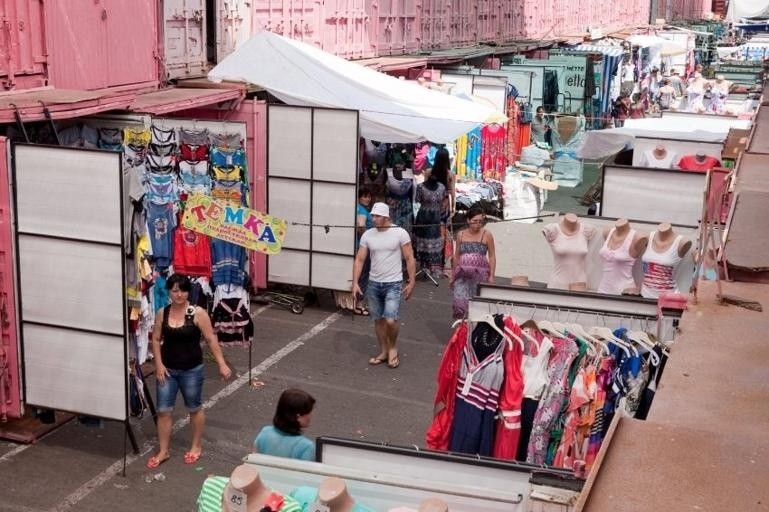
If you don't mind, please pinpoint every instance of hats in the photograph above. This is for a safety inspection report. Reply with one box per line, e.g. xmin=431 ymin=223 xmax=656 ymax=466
xmin=370 ymin=202 xmax=389 ymax=218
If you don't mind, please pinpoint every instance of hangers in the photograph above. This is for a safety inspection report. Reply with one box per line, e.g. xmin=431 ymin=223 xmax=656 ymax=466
xmin=148 ymin=118 xmax=245 ymax=211
xmin=448 ymin=299 xmax=673 ymax=366
xmin=70 ymin=121 xmax=147 ymax=152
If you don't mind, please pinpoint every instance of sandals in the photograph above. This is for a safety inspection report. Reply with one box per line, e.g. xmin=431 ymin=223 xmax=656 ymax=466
xmin=354 ymin=307 xmax=369 ymax=315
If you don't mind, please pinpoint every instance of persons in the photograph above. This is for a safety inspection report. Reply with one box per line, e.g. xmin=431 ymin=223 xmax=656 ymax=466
xmin=352 ymin=137 xmax=495 ymax=368
xmin=147 ymin=273 xmax=231 ymax=468
xmin=637 ymin=144 xmax=680 ymax=169
xmin=511 ymin=212 xmax=693 ymax=298
xmin=197 ymin=466 xmax=448 ymax=512
xmin=677 ymin=152 xmax=722 ymax=171
xmin=686 ymin=73 xmax=711 ymax=113
xmin=530 ymin=106 xmax=549 ymax=144
xmin=613 ymin=50 xmax=703 ymax=128
xmin=707 ymin=74 xmax=734 ymax=115
xmin=253 ymin=389 xmax=317 ymax=460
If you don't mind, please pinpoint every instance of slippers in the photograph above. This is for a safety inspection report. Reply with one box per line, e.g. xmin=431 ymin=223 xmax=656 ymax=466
xmin=147 ymin=457 xmax=160 ymax=470
xmin=183 ymin=452 xmax=201 ymax=464
xmin=368 ymin=352 xmax=400 ymax=367
